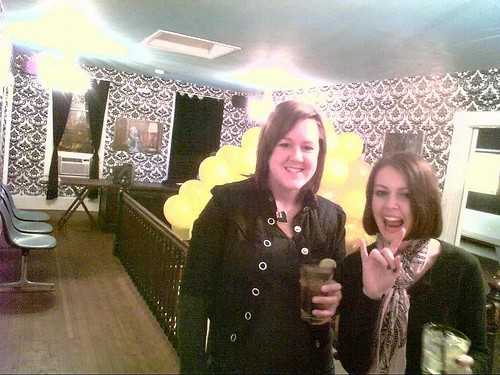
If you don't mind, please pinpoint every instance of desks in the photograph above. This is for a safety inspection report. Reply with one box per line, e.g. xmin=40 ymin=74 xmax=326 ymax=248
xmin=59 ymin=177 xmax=113 ymax=231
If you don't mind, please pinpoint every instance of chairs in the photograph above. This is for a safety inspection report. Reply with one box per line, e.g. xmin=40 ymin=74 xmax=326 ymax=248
xmin=0 ymin=183 xmax=57 ymax=290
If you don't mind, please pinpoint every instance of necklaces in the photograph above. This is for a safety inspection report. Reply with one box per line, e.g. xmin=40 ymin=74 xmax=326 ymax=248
xmin=275 ymin=203 xmax=298 ymax=225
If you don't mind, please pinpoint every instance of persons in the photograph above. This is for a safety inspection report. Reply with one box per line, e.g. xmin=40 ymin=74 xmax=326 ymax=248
xmin=176 ymin=100 xmax=347 ymax=375
xmin=337 ymin=152 xmax=488 ymax=375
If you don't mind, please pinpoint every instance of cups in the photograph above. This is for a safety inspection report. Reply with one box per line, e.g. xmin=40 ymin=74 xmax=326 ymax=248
xmin=420 ymin=324 xmax=471 ymax=375
xmin=299 ymin=264 xmax=334 ymax=323
